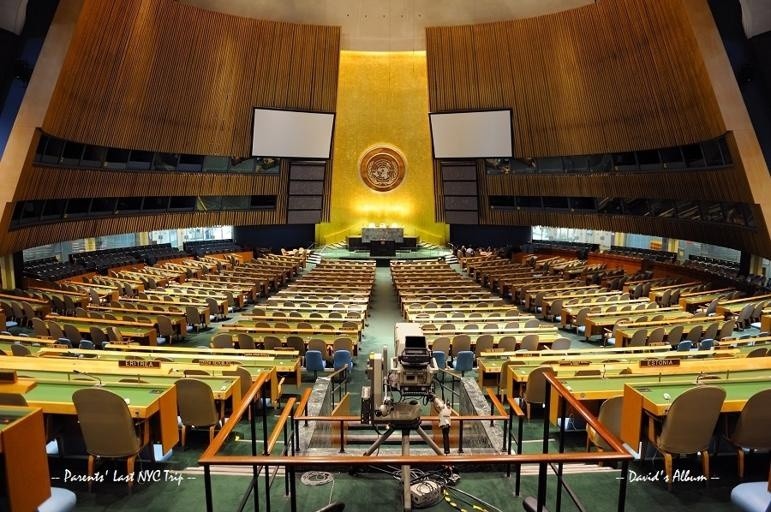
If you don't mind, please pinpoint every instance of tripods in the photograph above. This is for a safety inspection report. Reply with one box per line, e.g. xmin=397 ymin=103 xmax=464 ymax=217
xmin=348 ymin=427 xmax=447 ymax=512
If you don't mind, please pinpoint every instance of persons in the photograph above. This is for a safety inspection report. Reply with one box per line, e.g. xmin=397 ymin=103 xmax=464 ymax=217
xmin=460 ymin=244 xmax=499 ymax=258
